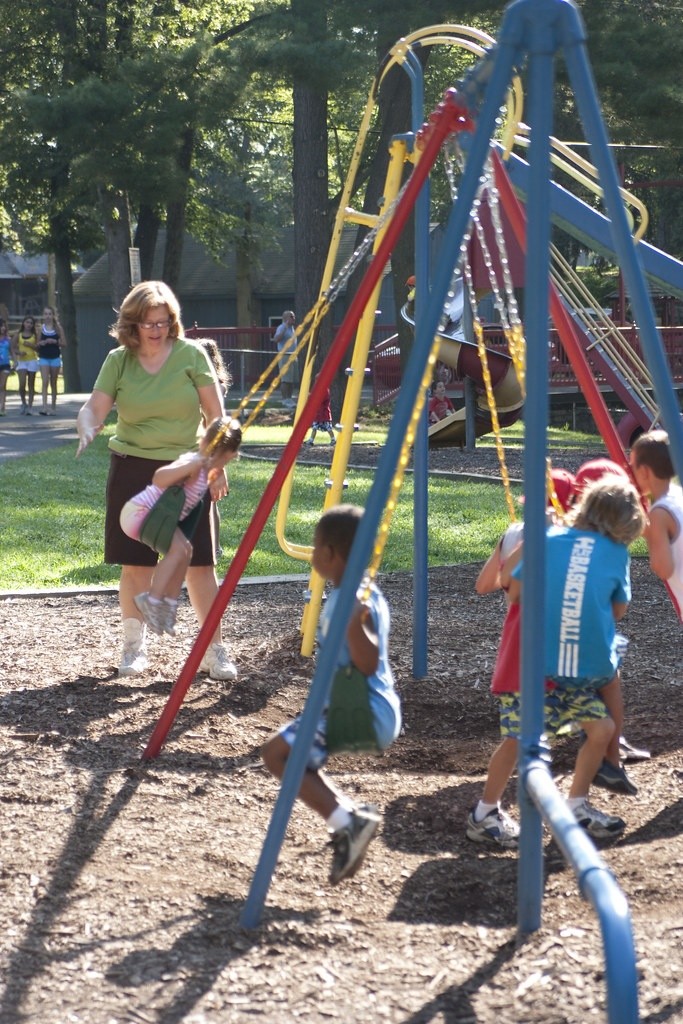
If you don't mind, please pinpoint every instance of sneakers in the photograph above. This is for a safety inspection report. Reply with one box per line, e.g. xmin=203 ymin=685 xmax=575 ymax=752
xmin=118 ymin=634 xmax=147 ymax=675
xmin=594 ymin=758 xmax=638 ymax=796
xmin=465 ymin=806 xmax=520 ymax=850
xmin=165 ymin=601 xmax=179 ymax=638
xmin=572 ymin=795 xmax=626 ymax=838
xmin=326 ymin=804 xmax=381 ymax=888
xmin=201 ymin=643 xmax=237 ymax=680
xmin=133 ymin=593 xmax=168 ymax=637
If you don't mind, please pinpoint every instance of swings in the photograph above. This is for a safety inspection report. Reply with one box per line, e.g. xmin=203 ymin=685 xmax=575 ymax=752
xmin=318 ymin=90 xmax=569 ymax=759
xmin=137 ymin=161 xmax=418 ymax=556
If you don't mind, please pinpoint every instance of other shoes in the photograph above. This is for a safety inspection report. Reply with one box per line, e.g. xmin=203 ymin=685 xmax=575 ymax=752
xmin=26 ymin=408 xmax=33 ymax=415
xmin=617 ymin=736 xmax=651 ymax=762
xmin=0 ymin=410 xmax=6 ymax=416
xmin=304 ymin=440 xmax=314 ymax=445
xmin=20 ymin=405 xmax=27 ymax=414
xmin=330 ymin=439 xmax=337 ymax=445
xmin=282 ymin=399 xmax=296 ymax=408
xmin=39 ymin=408 xmax=56 ymax=415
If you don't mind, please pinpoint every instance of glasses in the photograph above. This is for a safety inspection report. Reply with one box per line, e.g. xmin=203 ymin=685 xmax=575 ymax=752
xmin=287 ymin=316 xmax=296 ymax=319
xmin=137 ymin=319 xmax=172 ymax=328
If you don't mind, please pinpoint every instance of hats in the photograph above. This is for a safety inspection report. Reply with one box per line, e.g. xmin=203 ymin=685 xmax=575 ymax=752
xmin=405 ymin=275 xmax=415 ymax=285
xmin=575 ymin=458 xmax=630 ymax=502
xmin=517 ymin=468 xmax=579 ymax=512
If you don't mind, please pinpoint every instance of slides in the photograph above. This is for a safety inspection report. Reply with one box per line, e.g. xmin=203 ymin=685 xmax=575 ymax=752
xmin=399 ymin=275 xmax=528 ymax=448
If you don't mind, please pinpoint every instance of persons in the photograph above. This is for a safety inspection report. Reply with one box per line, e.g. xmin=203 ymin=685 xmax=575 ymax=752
xmin=0 ymin=318 xmax=18 ymax=416
xmin=36 ymin=306 xmax=67 ymax=416
xmin=428 ymin=382 xmax=456 ymax=425
xmin=405 ymin=276 xmax=416 ymax=301
xmin=76 ymin=281 xmax=239 ymax=680
xmin=273 ymin=310 xmax=300 ymax=408
xmin=12 ymin=316 xmax=39 ymax=415
xmin=303 ymin=374 xmax=335 ymax=446
xmin=195 ymin=339 xmax=231 ymax=434
xmin=467 ymin=430 xmax=683 ymax=847
xmin=263 ymin=507 xmax=403 ymax=887
xmin=120 ymin=416 xmax=242 ymax=635
